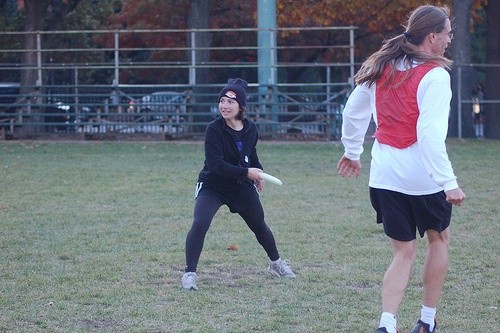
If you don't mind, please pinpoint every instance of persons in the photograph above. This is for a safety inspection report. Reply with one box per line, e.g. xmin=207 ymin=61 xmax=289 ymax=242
xmin=470 ymin=83 xmax=488 ymax=138
xmin=337 ymin=6 xmax=465 ymax=333
xmin=181 ymin=79 xmax=296 ymax=292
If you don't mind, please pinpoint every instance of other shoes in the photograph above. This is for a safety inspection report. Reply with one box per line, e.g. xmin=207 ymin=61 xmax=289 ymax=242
xmin=412 ymin=319 xmax=439 ymax=333
xmin=376 ymin=326 xmax=401 ymax=333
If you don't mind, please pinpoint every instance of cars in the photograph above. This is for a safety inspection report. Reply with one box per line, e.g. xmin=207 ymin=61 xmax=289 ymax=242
xmin=212 ymin=93 xmax=344 ymax=132
xmin=138 ymin=92 xmax=185 ymax=121
xmin=0 ymin=83 xmax=88 ymax=135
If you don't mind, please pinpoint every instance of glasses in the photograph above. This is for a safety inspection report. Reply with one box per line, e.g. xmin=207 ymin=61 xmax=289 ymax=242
xmin=435 ymin=33 xmax=454 ymax=39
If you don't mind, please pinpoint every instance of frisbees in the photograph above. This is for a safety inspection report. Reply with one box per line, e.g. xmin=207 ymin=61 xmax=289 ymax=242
xmin=260 ymin=172 xmax=283 ymax=186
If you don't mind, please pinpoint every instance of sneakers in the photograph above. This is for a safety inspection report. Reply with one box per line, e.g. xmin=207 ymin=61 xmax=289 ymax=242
xmin=181 ymin=272 xmax=199 ymax=291
xmin=267 ymin=258 xmax=297 ymax=281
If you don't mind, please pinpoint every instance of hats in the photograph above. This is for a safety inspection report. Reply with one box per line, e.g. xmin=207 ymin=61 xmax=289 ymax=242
xmin=218 ymin=78 xmax=249 ymax=109
